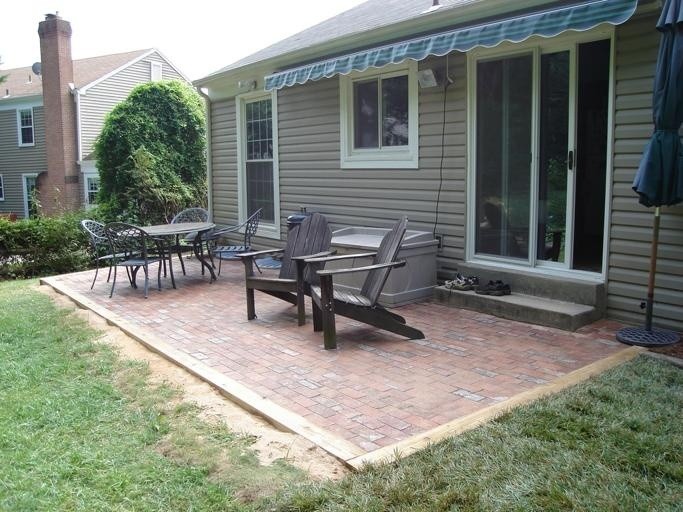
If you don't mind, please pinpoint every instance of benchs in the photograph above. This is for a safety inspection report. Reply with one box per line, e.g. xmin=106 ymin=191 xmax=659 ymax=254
xmin=324 ymin=226 xmax=439 ymax=309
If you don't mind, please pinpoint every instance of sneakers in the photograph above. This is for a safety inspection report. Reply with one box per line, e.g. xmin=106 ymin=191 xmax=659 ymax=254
xmin=476 ymin=280 xmax=511 ymax=296
xmin=444 ymin=274 xmax=480 ymax=291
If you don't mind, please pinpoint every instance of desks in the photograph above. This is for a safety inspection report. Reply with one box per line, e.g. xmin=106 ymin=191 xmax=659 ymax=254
xmin=117 ymin=222 xmax=216 ymax=292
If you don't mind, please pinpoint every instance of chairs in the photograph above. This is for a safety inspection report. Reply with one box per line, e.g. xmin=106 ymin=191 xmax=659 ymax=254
xmin=209 ymin=208 xmax=264 ymax=284
xmin=81 ymin=220 xmax=139 ymax=299
xmin=479 ymin=203 xmax=566 ymax=261
xmin=104 ymin=222 xmax=176 ymax=300
xmin=304 ymin=214 xmax=424 ymax=351
xmin=234 ymin=213 xmax=332 ymax=327
xmin=162 ymin=208 xmax=216 ymax=275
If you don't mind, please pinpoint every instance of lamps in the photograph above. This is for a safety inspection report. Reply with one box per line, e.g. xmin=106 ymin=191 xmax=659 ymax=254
xmin=416 ymin=68 xmax=438 ymax=89
xmin=237 ymin=79 xmax=257 ymax=90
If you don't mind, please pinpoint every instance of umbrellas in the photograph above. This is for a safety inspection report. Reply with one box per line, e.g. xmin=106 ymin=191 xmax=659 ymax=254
xmin=630 ymin=1 xmax=682 ymax=333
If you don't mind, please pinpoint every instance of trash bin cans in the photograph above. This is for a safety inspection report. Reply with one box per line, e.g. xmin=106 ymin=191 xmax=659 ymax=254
xmin=287 ymin=214 xmax=308 ymax=232
